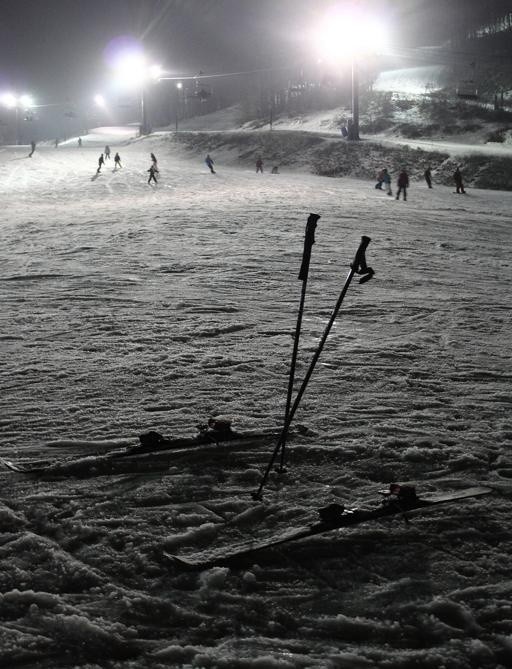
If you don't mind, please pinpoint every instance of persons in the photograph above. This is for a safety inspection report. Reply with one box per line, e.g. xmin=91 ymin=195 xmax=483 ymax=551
xmin=383 ymin=169 xmax=392 ymax=195
xmin=114 ymin=152 xmax=123 ymax=170
xmin=97 ymin=153 xmax=106 ymax=173
xmin=452 ymin=166 xmax=466 ymax=194
xmin=395 ymin=169 xmax=410 ymax=201
xmin=255 ymin=157 xmax=264 ymax=173
xmin=78 ymin=137 xmax=82 ymax=146
xmin=150 ymin=153 xmax=158 ymax=170
xmin=55 ymin=137 xmax=59 ymax=148
xmin=104 ymin=145 xmax=111 ymax=160
xmin=147 ymin=166 xmax=160 ymax=184
xmin=374 ymin=169 xmax=385 ymax=189
xmin=424 ymin=166 xmax=432 ymax=188
xmin=29 ymin=140 xmax=36 ymax=157
xmin=205 ymin=154 xmax=217 ymax=173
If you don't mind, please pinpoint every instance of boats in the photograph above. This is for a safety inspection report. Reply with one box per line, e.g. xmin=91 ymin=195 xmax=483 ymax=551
xmin=165 ymin=482 xmax=495 ymax=567
xmin=5 ymin=422 xmax=310 ymax=475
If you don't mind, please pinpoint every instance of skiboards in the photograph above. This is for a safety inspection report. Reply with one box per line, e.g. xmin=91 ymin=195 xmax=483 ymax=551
xmin=2 ymin=423 xmax=493 ymax=567
